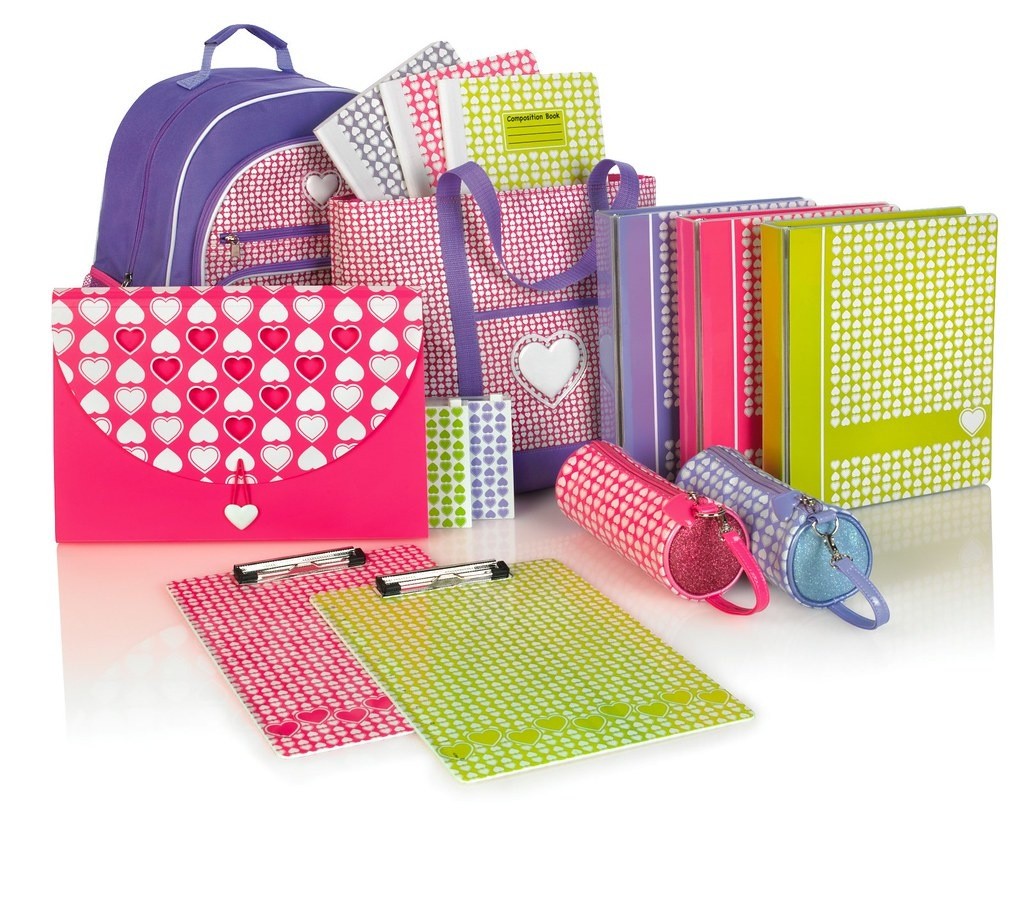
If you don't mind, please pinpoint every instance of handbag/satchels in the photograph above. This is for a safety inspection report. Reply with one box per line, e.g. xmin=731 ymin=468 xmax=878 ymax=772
xmin=328 ymin=159 xmax=656 ymax=494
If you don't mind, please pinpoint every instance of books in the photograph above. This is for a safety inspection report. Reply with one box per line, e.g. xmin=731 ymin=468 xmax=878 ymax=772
xmin=425 ymin=393 xmax=515 ymax=527
xmin=312 ymin=40 xmax=607 ymax=200
xmin=591 ymin=192 xmax=998 ymax=509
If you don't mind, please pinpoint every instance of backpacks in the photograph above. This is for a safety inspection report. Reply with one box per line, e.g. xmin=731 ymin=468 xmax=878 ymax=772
xmin=92 ymin=24 xmax=362 ymax=285
